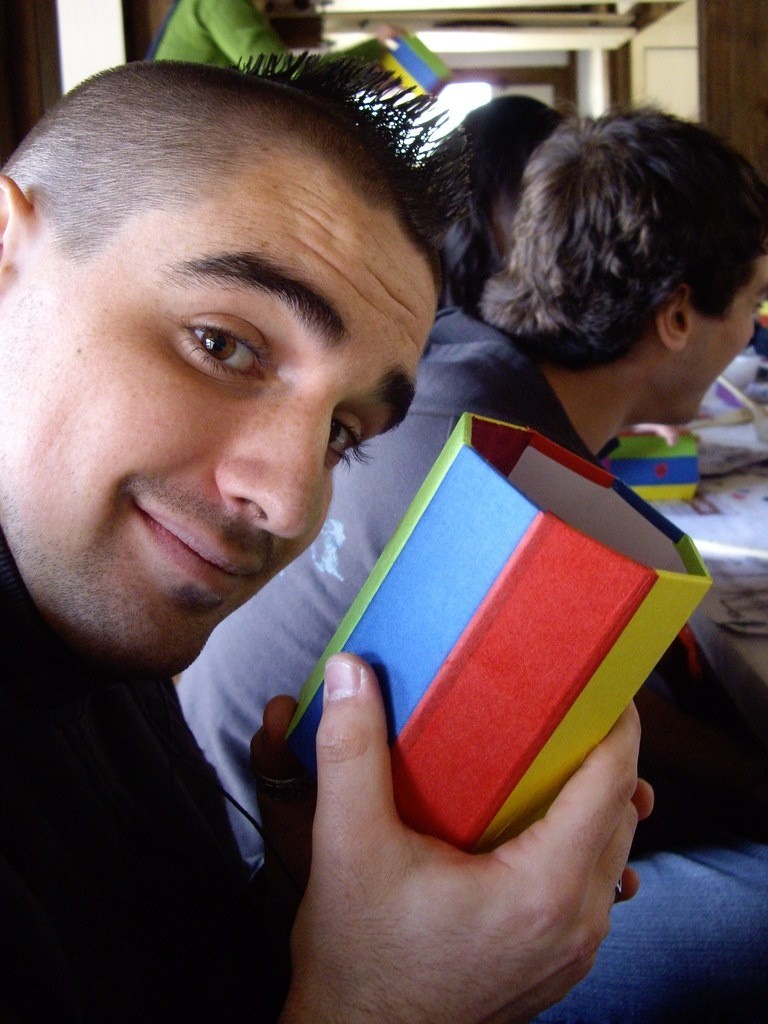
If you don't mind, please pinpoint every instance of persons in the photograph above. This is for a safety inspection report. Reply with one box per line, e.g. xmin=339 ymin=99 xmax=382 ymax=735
xmin=0 ymin=59 xmax=649 ymax=1024
xmin=152 ymin=0 xmax=768 ymax=1024
